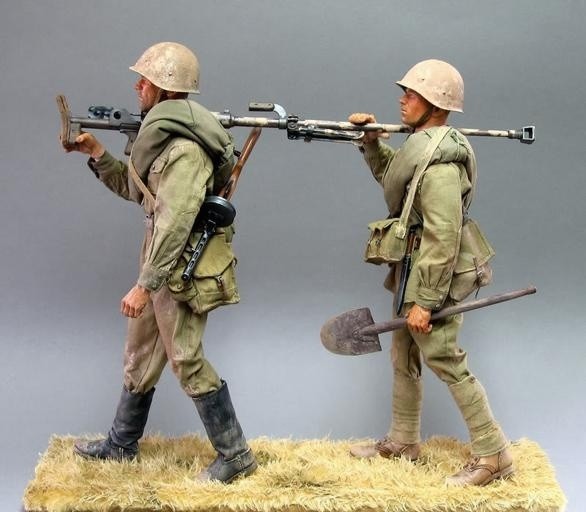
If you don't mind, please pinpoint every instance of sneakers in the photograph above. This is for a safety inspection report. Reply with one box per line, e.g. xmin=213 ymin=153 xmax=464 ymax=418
xmin=450 ymin=446 xmax=513 ymax=486
xmin=351 ymin=436 xmax=419 ymax=463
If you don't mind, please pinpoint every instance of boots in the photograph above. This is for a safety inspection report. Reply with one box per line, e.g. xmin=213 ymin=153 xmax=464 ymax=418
xmin=192 ymin=380 xmax=257 ymax=484
xmin=75 ymin=385 xmax=155 ymax=465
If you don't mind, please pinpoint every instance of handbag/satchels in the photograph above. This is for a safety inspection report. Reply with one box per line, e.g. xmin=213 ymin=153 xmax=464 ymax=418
xmin=449 ymin=219 xmax=495 ymax=302
xmin=167 ymin=234 xmax=241 ymax=315
xmin=364 ymin=218 xmax=407 ymax=265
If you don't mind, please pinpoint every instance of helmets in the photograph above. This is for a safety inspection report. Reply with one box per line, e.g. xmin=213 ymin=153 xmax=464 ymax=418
xmin=130 ymin=41 xmax=202 ymax=94
xmin=396 ymin=60 xmax=466 ymax=113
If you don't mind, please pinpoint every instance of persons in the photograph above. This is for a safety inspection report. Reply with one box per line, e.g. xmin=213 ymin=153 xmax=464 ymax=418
xmin=59 ymin=41 xmax=258 ymax=489
xmin=348 ymin=58 xmax=516 ymax=490
